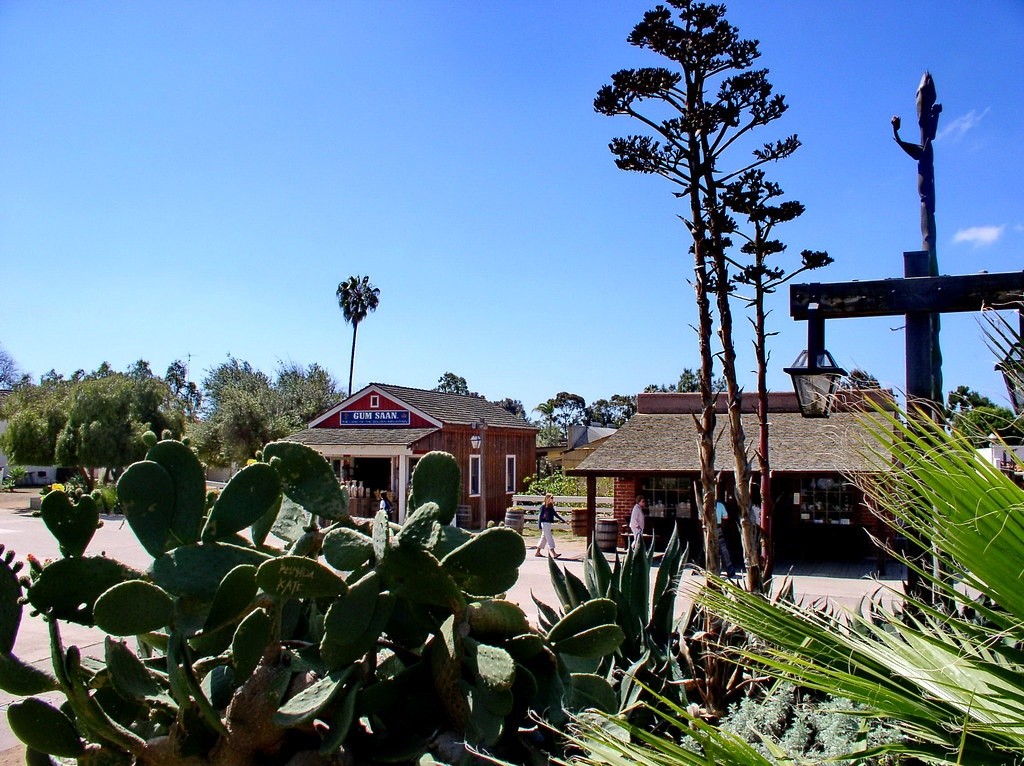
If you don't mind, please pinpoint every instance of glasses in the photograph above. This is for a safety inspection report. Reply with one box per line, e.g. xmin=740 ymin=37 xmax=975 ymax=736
xmin=550 ymin=497 xmax=553 ymax=499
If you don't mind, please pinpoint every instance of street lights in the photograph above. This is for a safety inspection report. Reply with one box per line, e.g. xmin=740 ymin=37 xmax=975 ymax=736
xmin=470 ymin=416 xmax=489 ymax=531
xmin=783 ymin=248 xmax=1024 ymax=630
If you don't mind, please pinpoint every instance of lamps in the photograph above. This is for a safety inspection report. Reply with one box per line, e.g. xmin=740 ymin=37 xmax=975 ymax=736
xmin=470 ymin=431 xmax=482 ymax=451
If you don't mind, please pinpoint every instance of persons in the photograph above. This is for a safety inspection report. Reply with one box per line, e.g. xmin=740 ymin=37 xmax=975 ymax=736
xmin=534 ymin=493 xmax=566 ymax=559
xmin=380 ymin=490 xmax=395 ymax=521
xmin=698 ymin=497 xmax=735 ymax=577
xmin=630 ymin=495 xmax=646 ymax=550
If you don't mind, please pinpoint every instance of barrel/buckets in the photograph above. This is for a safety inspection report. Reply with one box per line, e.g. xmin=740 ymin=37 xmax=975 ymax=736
xmin=597 ymin=519 xmax=618 ymax=552
xmin=505 ymin=508 xmax=524 ymax=535
xmin=456 ymin=505 xmax=472 ymax=529
xmin=571 ymin=508 xmax=588 ymax=536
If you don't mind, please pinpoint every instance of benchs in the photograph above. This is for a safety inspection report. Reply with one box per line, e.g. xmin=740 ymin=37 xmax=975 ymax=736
xmin=621 ymin=524 xmax=661 ymax=550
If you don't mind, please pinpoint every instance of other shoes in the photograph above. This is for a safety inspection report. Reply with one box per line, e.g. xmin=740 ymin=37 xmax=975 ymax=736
xmin=535 ymin=553 xmax=545 ymax=557
xmin=554 ymin=553 xmax=561 ymax=559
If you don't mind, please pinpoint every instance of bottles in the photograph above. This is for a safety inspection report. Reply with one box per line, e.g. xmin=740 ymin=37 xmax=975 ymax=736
xmin=345 ymin=480 xmax=364 ymax=498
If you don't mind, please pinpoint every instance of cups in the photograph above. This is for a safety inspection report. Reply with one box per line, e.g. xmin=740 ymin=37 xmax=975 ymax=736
xmin=366 ymin=488 xmax=370 ymax=497
